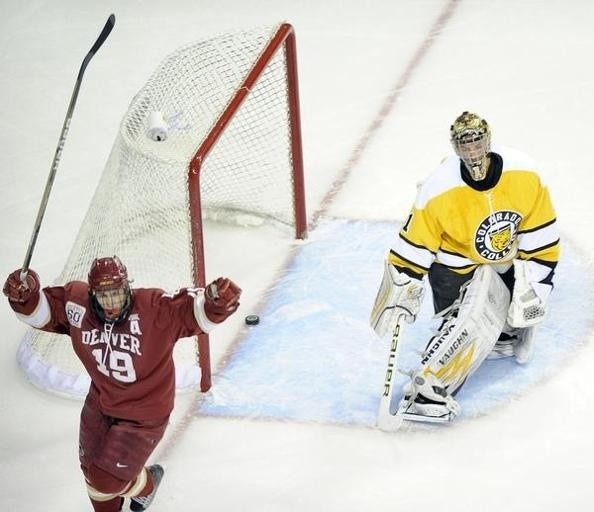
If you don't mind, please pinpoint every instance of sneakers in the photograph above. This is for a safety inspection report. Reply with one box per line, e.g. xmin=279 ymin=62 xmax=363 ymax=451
xmin=130 ymin=464 xmax=163 ymax=510
xmin=400 ymin=393 xmax=453 ymax=417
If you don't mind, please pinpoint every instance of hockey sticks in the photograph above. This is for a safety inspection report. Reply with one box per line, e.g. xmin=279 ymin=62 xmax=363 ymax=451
xmin=378 ymin=315 xmax=403 ymax=430
xmin=19 ymin=14 xmax=116 ymax=290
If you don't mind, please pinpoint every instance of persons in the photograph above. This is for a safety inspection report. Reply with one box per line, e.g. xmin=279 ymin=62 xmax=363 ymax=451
xmin=3 ymin=257 xmax=240 ymax=512
xmin=374 ymin=110 xmax=559 ymax=422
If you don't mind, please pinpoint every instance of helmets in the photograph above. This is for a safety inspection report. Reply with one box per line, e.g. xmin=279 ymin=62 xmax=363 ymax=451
xmin=88 ymin=257 xmax=132 ymax=322
xmin=450 ymin=113 xmax=491 ymax=182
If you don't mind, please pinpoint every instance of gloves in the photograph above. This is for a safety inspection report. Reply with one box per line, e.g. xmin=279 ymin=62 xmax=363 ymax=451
xmin=205 ymin=277 xmax=242 ymax=324
xmin=509 ymin=278 xmax=545 ymax=328
xmin=3 ymin=268 xmax=39 ymax=303
xmin=369 ymin=278 xmax=425 ymax=336
